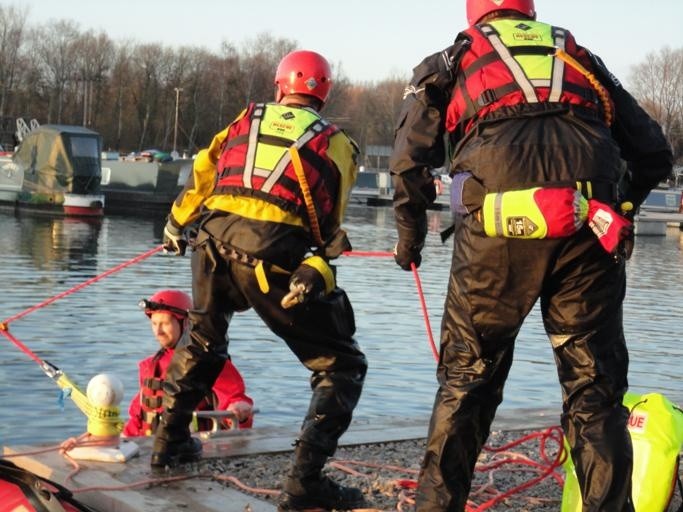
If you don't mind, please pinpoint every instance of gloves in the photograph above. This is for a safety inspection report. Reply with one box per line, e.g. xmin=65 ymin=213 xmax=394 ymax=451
xmin=394 ymin=239 xmax=425 ymax=270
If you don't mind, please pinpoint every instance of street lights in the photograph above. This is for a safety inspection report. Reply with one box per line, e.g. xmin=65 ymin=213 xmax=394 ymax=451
xmin=173 ymin=85 xmax=185 ymax=153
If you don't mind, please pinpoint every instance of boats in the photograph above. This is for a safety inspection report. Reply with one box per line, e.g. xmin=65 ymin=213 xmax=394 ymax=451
xmin=5 ymin=212 xmax=102 ymax=267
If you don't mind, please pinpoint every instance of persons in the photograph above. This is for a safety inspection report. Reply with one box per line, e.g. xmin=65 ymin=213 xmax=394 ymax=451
xmin=385 ymin=0 xmax=676 ymax=512
xmin=116 ymin=288 xmax=254 ymax=440
xmin=146 ymin=46 xmax=373 ymax=512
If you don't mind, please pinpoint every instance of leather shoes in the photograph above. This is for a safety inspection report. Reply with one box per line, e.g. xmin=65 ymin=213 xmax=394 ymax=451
xmin=151 ymin=438 xmax=202 ymax=466
xmin=281 ymin=478 xmax=363 ymax=507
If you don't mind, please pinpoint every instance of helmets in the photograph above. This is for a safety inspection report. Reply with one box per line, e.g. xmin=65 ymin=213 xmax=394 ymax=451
xmin=137 ymin=290 xmax=192 ymax=319
xmin=467 ymin=0 xmax=535 ymax=23
xmin=275 ymin=50 xmax=332 ymax=103
